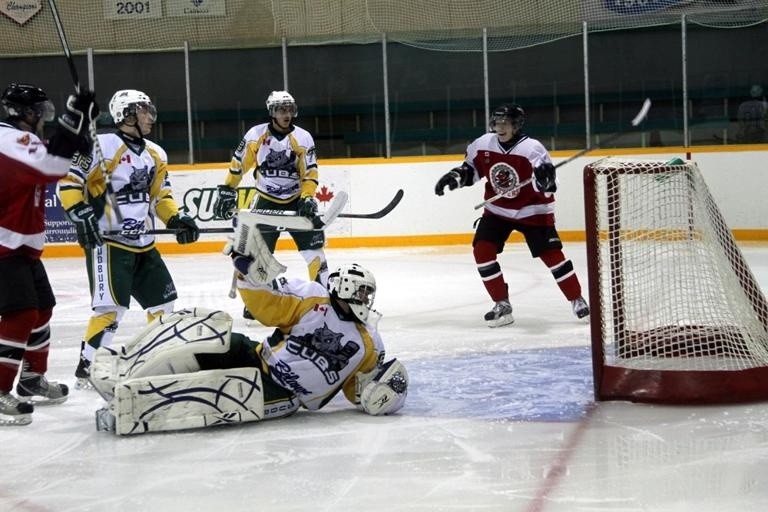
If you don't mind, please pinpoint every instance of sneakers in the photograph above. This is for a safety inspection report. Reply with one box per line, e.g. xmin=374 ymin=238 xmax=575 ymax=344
xmin=485 ymin=300 xmax=512 ymax=320
xmin=0 ymin=392 xmax=33 ymax=414
xmin=75 ymin=362 xmax=91 ymax=377
xmin=572 ymin=296 xmax=589 ymax=319
xmin=17 ymin=376 xmax=68 ymax=399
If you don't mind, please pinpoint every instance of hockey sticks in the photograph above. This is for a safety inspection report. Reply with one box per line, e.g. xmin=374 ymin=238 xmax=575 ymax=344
xmin=227 ymin=190 xmax=404 ymax=219
xmin=229 ymin=209 xmax=314 ymax=299
xmin=475 ymin=98 xmax=651 ymax=208
xmin=99 ymin=190 xmax=348 ymax=234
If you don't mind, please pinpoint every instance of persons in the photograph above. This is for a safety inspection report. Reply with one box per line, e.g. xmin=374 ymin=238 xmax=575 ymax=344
xmin=55 ymin=86 xmax=200 ymax=380
xmin=210 ymin=87 xmax=332 ymax=319
xmin=0 ymin=82 xmax=93 ymax=419
xmin=728 ymin=83 xmax=767 ymax=142
xmin=432 ymin=104 xmax=590 ymax=322
xmin=88 ymin=240 xmax=410 ymax=434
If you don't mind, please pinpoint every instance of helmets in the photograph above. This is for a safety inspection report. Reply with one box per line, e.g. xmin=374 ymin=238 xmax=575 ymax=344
xmin=109 ymin=89 xmax=157 ymax=128
xmin=0 ymin=81 xmax=55 ymax=133
xmin=360 ymin=359 xmax=408 ymax=416
xmin=326 ymin=263 xmax=383 ymax=330
xmin=489 ymin=104 xmax=528 ymax=130
xmin=266 ymin=90 xmax=299 ymax=118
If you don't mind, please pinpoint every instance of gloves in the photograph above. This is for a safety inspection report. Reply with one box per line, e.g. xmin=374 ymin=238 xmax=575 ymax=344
xmin=435 ymin=167 xmax=467 ymax=195
xmin=66 ymin=201 xmax=103 ymax=250
xmin=57 ymin=90 xmax=101 ymax=136
xmin=533 ymin=163 xmax=555 ymax=190
xmin=168 ymin=211 xmax=198 ymax=244
xmin=298 ymin=197 xmax=318 ymax=218
xmin=213 ymin=184 xmax=237 ymax=219
xmin=222 ymin=241 xmax=261 ymax=284
